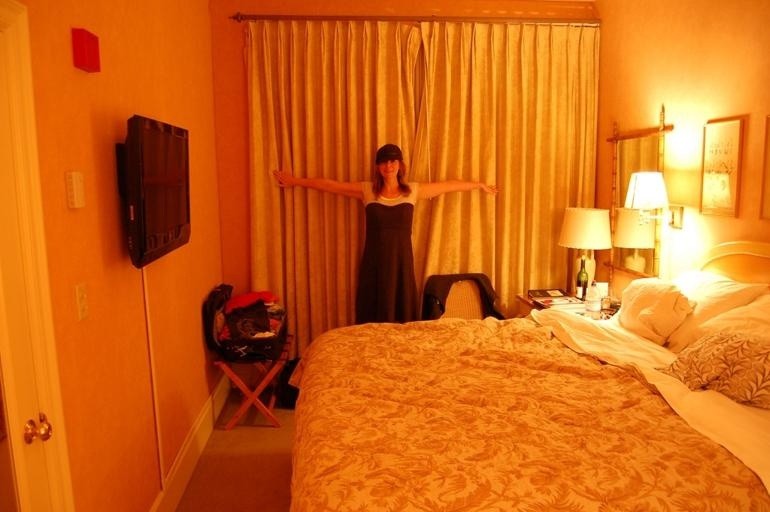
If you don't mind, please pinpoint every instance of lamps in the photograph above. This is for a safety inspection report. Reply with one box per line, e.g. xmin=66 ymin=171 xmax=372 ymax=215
xmin=625 ymin=170 xmax=684 ymax=230
xmin=557 ymin=206 xmax=613 ymax=303
xmin=612 ymin=208 xmax=657 ymax=275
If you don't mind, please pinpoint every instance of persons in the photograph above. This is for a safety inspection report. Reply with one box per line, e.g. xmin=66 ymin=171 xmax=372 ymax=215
xmin=272 ymin=143 xmax=507 ymax=324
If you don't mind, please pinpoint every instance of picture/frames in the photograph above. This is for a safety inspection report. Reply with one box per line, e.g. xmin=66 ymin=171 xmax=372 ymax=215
xmin=700 ymin=112 xmax=752 ymax=220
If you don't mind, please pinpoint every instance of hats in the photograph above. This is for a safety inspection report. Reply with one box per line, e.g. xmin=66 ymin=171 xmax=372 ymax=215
xmin=376 ymin=143 xmax=403 ymax=161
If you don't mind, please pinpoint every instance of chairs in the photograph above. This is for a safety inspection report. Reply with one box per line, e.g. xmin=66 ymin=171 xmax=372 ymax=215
xmin=422 ymin=273 xmax=505 ymax=322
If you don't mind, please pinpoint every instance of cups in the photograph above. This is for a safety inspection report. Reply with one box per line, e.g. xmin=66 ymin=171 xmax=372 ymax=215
xmin=601 ymin=296 xmax=611 ymax=310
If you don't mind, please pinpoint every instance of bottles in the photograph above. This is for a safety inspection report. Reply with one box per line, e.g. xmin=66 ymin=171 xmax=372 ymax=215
xmin=586 ymin=280 xmax=601 ymax=320
xmin=575 ymin=255 xmax=588 ymax=302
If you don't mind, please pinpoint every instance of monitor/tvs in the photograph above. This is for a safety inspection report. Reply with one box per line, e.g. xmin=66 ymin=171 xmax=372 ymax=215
xmin=126 ymin=114 xmax=190 ymax=269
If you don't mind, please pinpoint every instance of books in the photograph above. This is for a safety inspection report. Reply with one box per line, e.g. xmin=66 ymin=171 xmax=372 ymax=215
xmin=527 ymin=288 xmax=587 ymax=314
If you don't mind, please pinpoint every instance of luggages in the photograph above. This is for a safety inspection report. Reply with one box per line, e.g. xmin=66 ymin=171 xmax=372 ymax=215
xmin=203 ymin=282 xmax=288 ymax=360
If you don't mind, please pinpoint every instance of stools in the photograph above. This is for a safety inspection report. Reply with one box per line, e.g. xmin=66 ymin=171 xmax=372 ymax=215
xmin=208 ymin=334 xmax=295 ymax=432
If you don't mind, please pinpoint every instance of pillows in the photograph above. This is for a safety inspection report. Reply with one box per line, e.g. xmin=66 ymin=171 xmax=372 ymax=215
xmin=618 ymin=267 xmax=769 ymax=411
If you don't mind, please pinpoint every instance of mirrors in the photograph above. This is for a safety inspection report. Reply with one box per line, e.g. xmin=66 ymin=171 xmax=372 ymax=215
xmin=602 ymin=102 xmax=673 ymax=279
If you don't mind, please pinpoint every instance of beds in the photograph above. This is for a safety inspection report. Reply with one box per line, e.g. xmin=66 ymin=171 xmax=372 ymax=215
xmin=290 ymin=241 xmax=770 ymax=512
